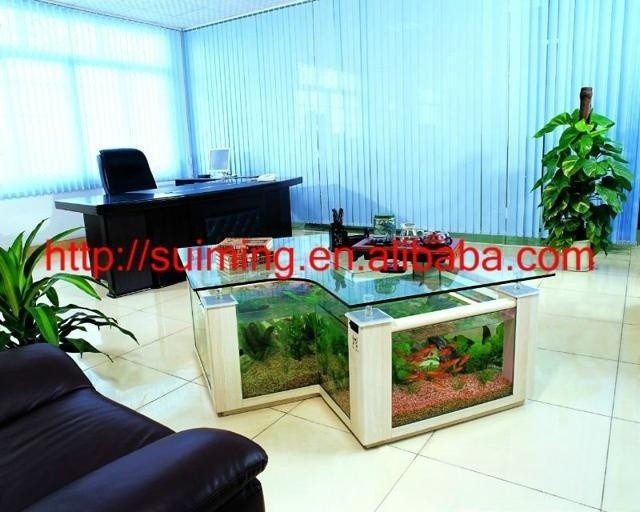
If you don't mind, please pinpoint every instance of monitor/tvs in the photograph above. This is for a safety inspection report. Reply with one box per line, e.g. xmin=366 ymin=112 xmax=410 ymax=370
xmin=208 ymin=148 xmax=231 ymax=179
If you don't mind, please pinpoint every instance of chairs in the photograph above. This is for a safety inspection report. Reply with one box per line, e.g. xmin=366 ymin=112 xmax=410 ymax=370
xmin=96 ymin=148 xmax=158 ymax=194
xmin=0 ymin=342 xmax=268 ymax=512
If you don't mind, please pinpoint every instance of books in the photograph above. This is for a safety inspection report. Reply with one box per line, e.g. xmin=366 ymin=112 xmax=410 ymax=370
xmin=330 ymin=255 xmax=414 ymax=283
xmin=213 ymin=235 xmax=277 ymax=271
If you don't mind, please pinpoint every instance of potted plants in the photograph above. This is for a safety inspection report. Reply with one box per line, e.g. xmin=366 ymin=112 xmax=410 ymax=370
xmin=528 ymin=87 xmax=633 ymax=272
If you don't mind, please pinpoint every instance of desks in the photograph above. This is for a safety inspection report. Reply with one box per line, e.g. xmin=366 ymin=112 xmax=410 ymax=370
xmin=55 ymin=176 xmax=303 ymax=299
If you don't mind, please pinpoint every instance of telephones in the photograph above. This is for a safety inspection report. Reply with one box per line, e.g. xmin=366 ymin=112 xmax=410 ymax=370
xmin=257 ymin=174 xmax=276 ymax=181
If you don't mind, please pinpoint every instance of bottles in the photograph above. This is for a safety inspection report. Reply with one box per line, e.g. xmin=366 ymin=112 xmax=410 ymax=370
xmin=374 ymin=214 xmax=396 ymax=242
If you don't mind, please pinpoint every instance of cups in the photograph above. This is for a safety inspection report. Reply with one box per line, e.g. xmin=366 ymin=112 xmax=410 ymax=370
xmin=373 ymin=235 xmax=387 ymax=246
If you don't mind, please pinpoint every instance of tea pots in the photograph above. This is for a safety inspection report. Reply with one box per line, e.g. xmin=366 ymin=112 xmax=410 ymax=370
xmin=399 ymin=223 xmax=453 ymax=247
